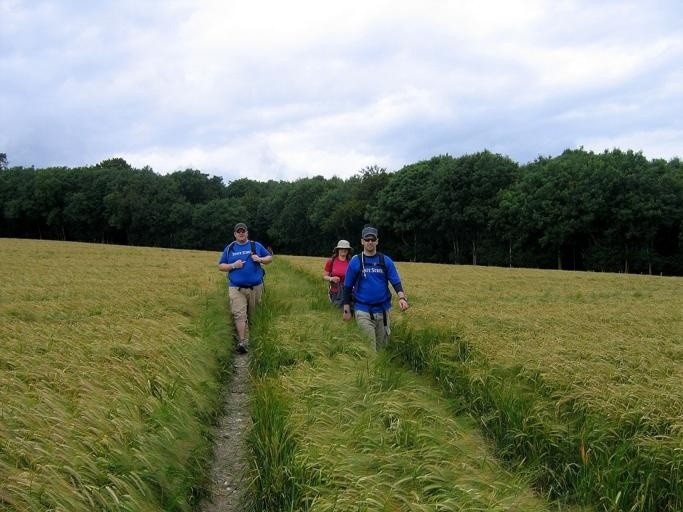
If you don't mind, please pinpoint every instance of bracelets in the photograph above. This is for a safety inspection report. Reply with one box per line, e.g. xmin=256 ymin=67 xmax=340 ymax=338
xmin=259 ymin=257 xmax=263 ymax=263
xmin=329 ymin=277 xmax=332 ymax=282
xmin=232 ymin=263 xmax=235 ymax=269
xmin=398 ymin=297 xmax=407 ymax=301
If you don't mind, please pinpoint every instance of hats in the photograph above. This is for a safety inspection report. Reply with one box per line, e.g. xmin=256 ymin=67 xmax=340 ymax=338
xmin=234 ymin=222 xmax=247 ymax=231
xmin=335 ymin=239 xmax=352 ymax=250
xmin=361 ymin=226 xmax=377 ymax=239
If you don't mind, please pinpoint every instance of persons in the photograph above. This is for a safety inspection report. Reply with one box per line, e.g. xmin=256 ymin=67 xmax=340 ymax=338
xmin=341 ymin=225 xmax=409 ymax=373
xmin=322 ymin=239 xmax=354 ymax=315
xmin=216 ymin=222 xmax=273 ymax=352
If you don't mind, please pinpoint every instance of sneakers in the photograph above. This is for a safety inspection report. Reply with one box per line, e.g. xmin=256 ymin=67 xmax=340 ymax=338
xmin=236 ymin=341 xmax=249 ymax=354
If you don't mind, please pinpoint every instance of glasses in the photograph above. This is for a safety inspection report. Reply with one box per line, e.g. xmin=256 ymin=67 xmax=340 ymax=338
xmin=364 ymin=238 xmax=376 ymax=243
